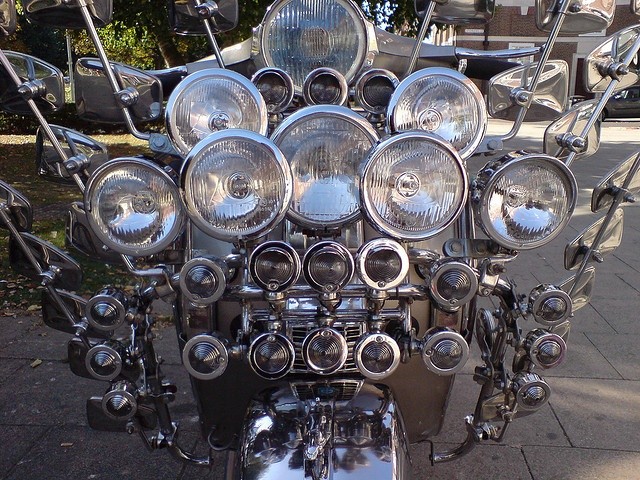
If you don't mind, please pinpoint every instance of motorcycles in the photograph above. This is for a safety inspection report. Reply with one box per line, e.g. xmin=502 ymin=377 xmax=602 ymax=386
xmin=0 ymin=0 xmax=640 ymax=478
